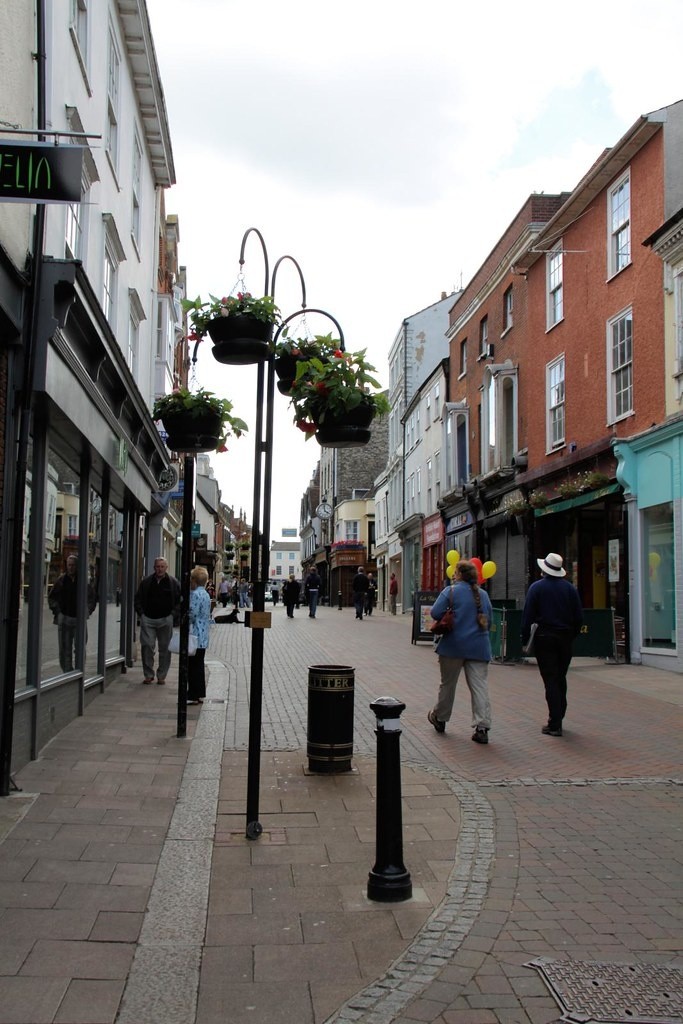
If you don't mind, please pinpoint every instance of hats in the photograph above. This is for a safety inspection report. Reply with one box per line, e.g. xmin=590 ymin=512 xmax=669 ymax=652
xmin=537 ymin=553 xmax=567 ymax=577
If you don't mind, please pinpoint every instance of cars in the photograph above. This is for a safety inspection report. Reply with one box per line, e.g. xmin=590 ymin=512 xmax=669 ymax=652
xmin=265 ymin=592 xmax=272 ymax=601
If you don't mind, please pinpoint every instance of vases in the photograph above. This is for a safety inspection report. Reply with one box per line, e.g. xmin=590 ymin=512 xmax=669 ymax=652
xmin=589 ymin=481 xmax=606 ymax=489
xmin=160 ymin=407 xmax=218 ymax=451
xmin=273 ymin=354 xmax=330 ymax=396
xmin=208 ymin=317 xmax=272 ymax=365
xmin=312 ymin=393 xmax=373 ymax=447
xmin=532 ymin=501 xmax=547 ymax=510
xmin=560 ymin=491 xmax=576 ymax=499
xmin=511 ymin=509 xmax=526 ymax=517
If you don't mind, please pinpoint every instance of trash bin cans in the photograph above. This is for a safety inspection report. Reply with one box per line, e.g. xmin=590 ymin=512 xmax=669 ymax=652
xmin=308 ymin=665 xmax=356 ymax=774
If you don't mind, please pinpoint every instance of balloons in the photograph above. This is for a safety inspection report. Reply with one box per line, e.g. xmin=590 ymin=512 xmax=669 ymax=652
xmin=445 ymin=549 xmax=496 ymax=586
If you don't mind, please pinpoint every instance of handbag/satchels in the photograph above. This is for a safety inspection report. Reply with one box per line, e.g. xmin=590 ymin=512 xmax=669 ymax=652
xmin=430 ymin=586 xmax=455 ymax=636
xmin=168 ymin=623 xmax=198 ymax=656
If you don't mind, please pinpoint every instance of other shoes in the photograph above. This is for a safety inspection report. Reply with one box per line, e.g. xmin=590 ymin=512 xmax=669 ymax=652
xmin=187 ymin=698 xmax=203 ymax=705
xmin=157 ymin=678 xmax=165 ymax=685
xmin=143 ymin=677 xmax=153 ymax=684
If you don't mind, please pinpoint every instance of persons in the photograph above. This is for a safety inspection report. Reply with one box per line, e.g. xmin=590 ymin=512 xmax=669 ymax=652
xmin=523 ymin=553 xmax=579 ymax=737
xmin=427 ymin=561 xmax=494 ymax=744
xmin=217 ymin=566 xmax=377 ymax=621
xmin=47 ymin=554 xmax=96 ymax=676
xmin=135 ymin=557 xmax=180 ymax=684
xmin=389 ymin=573 xmax=398 ymax=616
xmin=186 ymin=566 xmax=210 ymax=705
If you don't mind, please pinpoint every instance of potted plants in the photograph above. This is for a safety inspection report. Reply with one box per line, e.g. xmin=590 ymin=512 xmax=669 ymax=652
xmin=242 ymin=542 xmax=250 ymax=550
xmin=240 ymin=552 xmax=248 ymax=561
xmin=225 ymin=542 xmax=234 ymax=551
xmin=226 ymin=552 xmax=235 ymax=560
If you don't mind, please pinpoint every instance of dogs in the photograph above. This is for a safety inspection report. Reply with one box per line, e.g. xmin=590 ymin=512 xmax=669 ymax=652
xmin=214 ymin=609 xmax=246 ymax=623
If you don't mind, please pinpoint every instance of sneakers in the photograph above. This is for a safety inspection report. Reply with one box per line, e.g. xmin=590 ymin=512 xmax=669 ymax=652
xmin=428 ymin=710 xmax=446 ymax=732
xmin=472 ymin=728 xmax=489 ymax=744
xmin=542 ymin=724 xmax=562 ymax=736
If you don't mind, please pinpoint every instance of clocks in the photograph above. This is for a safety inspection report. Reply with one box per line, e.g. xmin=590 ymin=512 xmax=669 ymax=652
xmin=90 ymin=498 xmax=101 ymax=515
xmin=315 ymin=503 xmax=333 ymax=519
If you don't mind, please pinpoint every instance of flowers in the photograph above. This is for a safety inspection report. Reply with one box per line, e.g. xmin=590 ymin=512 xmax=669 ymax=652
xmin=272 ymin=327 xmax=390 ymax=442
xmin=503 ymin=470 xmax=609 ymax=519
xmin=152 ymin=385 xmax=248 ymax=454
xmin=179 ymin=294 xmax=287 ymax=341
